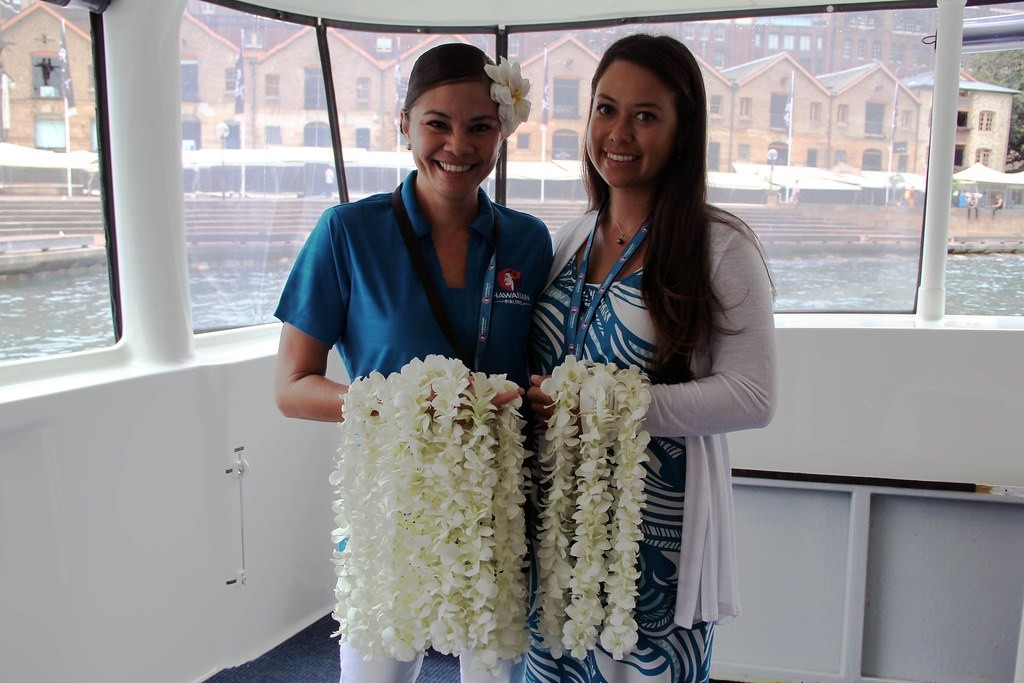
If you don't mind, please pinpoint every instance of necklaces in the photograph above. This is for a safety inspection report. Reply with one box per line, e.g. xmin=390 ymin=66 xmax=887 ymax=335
xmin=606 ymin=201 xmax=649 ymax=245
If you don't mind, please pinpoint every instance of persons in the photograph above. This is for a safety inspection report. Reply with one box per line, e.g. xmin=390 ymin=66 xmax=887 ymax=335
xmin=272 ymin=44 xmax=556 ymax=683
xmin=324 ymin=166 xmax=335 ymax=200
xmin=792 ymin=179 xmax=801 ymax=208
xmin=907 ymin=184 xmax=915 ymax=210
xmin=958 ymin=189 xmax=1006 ymax=218
xmin=525 ymin=34 xmax=779 ymax=683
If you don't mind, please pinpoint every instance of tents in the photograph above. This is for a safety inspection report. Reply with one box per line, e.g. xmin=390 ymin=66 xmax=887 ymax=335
xmin=953 ymin=162 xmax=1023 ymax=208
